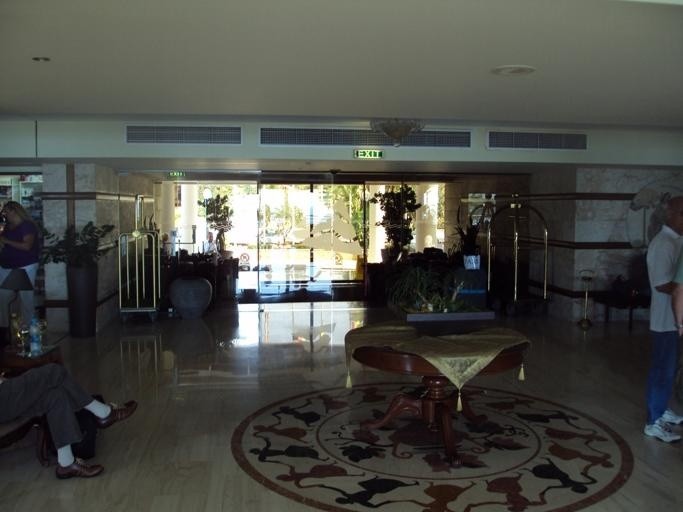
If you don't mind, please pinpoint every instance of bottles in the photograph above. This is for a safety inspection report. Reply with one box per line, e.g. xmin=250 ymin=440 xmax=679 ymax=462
xmin=29 ymin=319 xmax=41 ymax=351
xmin=9 ymin=313 xmax=19 ymax=348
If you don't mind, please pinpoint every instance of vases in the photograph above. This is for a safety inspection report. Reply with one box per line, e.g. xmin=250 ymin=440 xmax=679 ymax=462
xmin=168 ymin=275 xmax=213 ymax=320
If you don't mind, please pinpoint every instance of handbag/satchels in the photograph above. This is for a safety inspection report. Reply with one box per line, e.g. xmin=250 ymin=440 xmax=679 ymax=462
xmin=45 ymin=394 xmax=106 ymax=466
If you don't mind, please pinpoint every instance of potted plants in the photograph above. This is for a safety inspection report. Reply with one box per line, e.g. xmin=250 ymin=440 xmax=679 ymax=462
xmin=197 ymin=192 xmax=235 ymax=251
xmin=382 ymin=262 xmax=496 ymax=336
xmin=32 ymin=220 xmax=118 ymax=338
xmin=368 ymin=183 xmax=423 ymax=264
xmin=454 ymin=199 xmax=486 ymax=269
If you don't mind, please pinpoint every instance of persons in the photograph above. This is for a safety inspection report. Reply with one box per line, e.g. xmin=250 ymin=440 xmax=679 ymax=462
xmin=0 ymin=201 xmax=43 ymax=329
xmin=644 ymin=196 xmax=683 ymax=443
xmin=198 ymin=232 xmax=218 ymax=255
xmin=0 ymin=352 xmax=140 ymax=478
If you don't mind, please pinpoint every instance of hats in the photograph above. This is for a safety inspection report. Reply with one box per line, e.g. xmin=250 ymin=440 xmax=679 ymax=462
xmin=668 ymin=196 xmax=683 ymax=209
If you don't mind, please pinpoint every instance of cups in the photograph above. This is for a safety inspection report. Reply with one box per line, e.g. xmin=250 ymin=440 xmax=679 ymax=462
xmin=36 ymin=320 xmax=48 ymax=337
xmin=15 ymin=327 xmax=30 ymax=357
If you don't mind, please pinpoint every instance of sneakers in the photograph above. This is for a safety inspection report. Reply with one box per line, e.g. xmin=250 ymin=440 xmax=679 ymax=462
xmin=644 ymin=416 xmax=683 ymax=443
xmin=56 ymin=457 xmax=104 ymax=479
xmin=660 ymin=409 xmax=683 ymax=425
xmin=97 ymin=400 xmax=137 ymax=429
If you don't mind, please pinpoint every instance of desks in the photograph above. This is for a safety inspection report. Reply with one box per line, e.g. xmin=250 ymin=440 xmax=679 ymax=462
xmin=345 ymin=325 xmax=531 ymax=468
xmin=593 ymin=290 xmax=651 ymax=335
